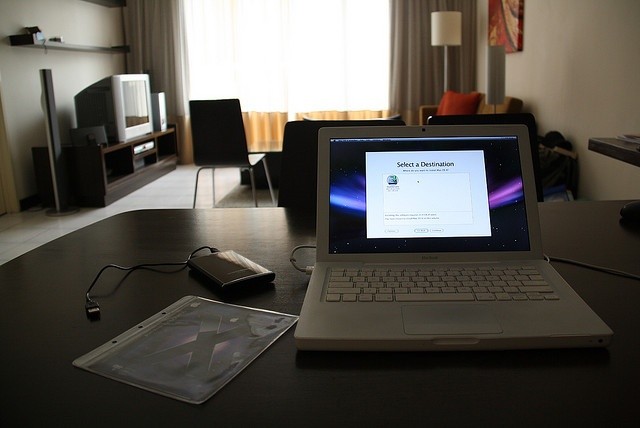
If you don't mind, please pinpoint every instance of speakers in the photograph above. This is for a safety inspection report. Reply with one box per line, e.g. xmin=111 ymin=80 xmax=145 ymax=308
xmin=149 ymin=92 xmax=168 ymax=132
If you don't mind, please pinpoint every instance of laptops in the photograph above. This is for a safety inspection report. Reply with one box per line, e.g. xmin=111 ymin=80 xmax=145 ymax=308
xmin=291 ymin=124 xmax=614 ymax=352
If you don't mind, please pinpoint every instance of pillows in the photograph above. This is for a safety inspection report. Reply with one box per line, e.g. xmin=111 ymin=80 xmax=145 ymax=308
xmin=436 ymin=92 xmax=481 ymax=115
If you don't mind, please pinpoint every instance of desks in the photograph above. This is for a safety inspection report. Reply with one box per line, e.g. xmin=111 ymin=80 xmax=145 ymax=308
xmin=0 ymin=198 xmax=640 ymax=428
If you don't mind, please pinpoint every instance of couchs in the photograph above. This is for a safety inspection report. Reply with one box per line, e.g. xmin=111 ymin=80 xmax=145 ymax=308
xmin=418 ymin=91 xmax=523 ymax=125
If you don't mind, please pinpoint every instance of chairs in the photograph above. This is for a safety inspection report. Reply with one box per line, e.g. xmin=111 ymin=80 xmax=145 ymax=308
xmin=189 ymin=99 xmax=276 ymax=207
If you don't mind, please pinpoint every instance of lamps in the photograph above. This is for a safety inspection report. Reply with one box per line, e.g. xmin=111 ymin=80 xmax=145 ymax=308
xmin=431 ymin=10 xmax=463 ymax=91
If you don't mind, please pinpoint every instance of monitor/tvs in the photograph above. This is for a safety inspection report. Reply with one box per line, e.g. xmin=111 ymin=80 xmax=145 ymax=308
xmin=73 ymin=74 xmax=153 ymax=143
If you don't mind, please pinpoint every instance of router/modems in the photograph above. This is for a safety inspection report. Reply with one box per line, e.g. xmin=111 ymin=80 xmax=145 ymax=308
xmin=188 ymin=249 xmax=276 ymax=297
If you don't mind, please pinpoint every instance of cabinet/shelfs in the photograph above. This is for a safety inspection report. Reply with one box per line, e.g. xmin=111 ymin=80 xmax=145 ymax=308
xmin=31 ymin=122 xmax=180 ymax=209
